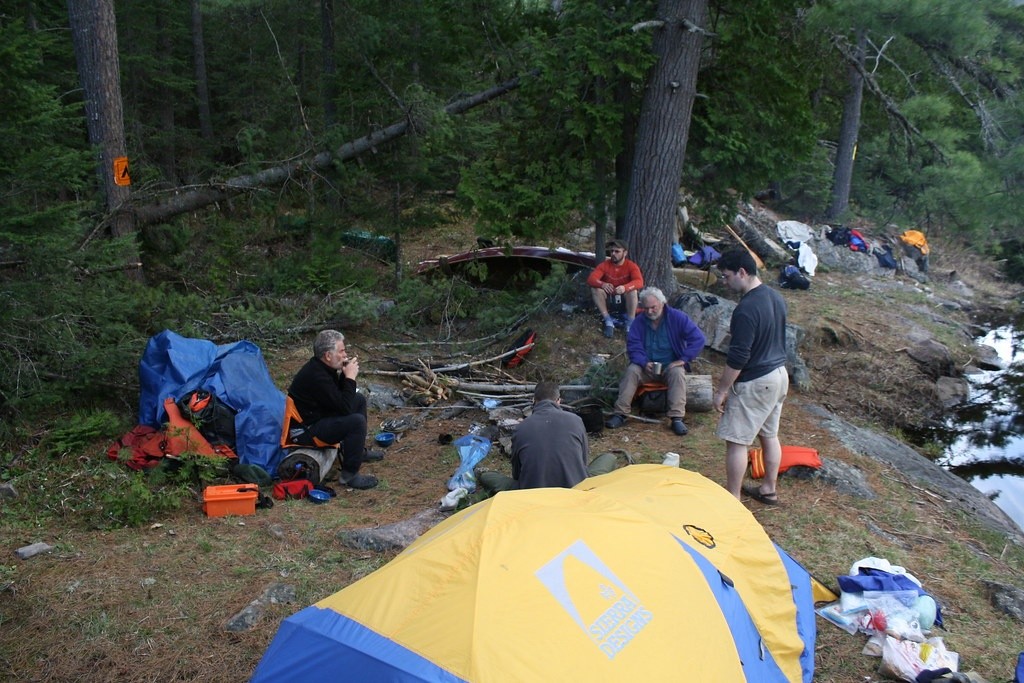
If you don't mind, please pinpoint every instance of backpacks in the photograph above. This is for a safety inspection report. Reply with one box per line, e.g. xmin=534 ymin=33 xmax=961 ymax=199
xmin=503 ymin=330 xmax=536 ymax=369
xmin=779 ymin=263 xmax=810 ymax=290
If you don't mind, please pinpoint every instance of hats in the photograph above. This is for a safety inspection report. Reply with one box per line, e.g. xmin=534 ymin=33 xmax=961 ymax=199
xmin=608 ymin=239 xmax=628 ymax=250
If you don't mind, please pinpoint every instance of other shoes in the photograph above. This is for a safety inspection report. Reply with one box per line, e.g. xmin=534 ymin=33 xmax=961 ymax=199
xmin=605 ymin=414 xmax=630 ymax=429
xmin=340 ymin=472 xmax=378 ymax=489
xmin=671 ymin=420 xmax=688 ymax=435
xmin=605 ymin=323 xmax=614 ymax=338
xmin=360 ymin=447 xmax=384 ymax=463
xmin=625 ymin=332 xmax=631 ymax=342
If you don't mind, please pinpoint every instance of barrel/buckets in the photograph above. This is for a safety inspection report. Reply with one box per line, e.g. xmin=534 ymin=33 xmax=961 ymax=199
xmin=575 ymin=404 xmax=604 ymax=432
xmin=671 ymin=244 xmax=687 ymax=267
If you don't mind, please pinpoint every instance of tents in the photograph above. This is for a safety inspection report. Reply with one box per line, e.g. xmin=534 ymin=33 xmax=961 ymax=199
xmin=249 ymin=464 xmax=840 ymax=683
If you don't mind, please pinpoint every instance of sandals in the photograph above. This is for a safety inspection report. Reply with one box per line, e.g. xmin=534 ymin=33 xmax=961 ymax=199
xmin=742 ymin=484 xmax=777 ymax=505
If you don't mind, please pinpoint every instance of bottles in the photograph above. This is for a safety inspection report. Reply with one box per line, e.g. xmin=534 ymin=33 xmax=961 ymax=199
xmin=614 ymin=288 xmax=621 ymax=304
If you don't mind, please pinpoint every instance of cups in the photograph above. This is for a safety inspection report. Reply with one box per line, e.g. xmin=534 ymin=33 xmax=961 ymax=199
xmin=651 ymin=362 xmax=662 ymax=374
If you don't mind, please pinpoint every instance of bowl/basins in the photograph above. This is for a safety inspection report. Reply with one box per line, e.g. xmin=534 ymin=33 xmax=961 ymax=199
xmin=308 ymin=490 xmax=331 ymax=504
xmin=375 ymin=433 xmax=394 ymax=447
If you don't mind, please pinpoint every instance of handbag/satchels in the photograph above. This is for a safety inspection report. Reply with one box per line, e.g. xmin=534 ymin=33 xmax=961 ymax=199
xmin=273 ymin=468 xmax=313 ymax=501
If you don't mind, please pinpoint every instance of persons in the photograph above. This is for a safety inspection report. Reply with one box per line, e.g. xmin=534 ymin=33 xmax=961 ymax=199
xmin=481 ymin=381 xmax=615 ymax=493
xmin=588 ymin=240 xmax=643 ymax=337
xmin=714 ymin=250 xmax=789 ymax=503
xmin=606 ymin=287 xmax=704 ymax=435
xmin=280 ymin=331 xmax=384 ymax=490
